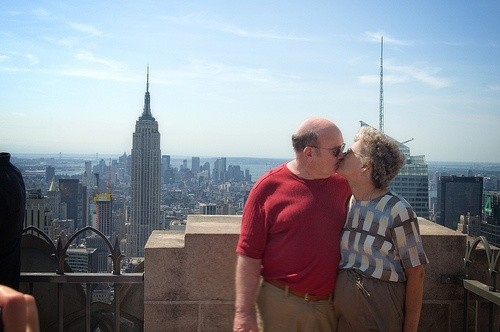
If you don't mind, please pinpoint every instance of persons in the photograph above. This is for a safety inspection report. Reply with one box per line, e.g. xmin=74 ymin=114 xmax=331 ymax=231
xmin=333 ymin=124 xmax=430 ymax=332
xmin=233 ymin=117 xmax=354 ymax=332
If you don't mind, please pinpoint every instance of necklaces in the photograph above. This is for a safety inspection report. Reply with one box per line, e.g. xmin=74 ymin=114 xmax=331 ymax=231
xmin=346 ymin=189 xmax=376 ymax=232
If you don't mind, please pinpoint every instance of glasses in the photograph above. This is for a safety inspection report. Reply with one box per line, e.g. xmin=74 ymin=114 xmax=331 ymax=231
xmin=303 ymin=143 xmax=346 ymax=157
xmin=347 ymin=147 xmax=363 ymax=158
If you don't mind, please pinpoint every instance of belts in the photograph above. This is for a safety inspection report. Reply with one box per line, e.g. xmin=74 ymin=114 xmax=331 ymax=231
xmin=263 ymin=279 xmax=334 ymax=302
